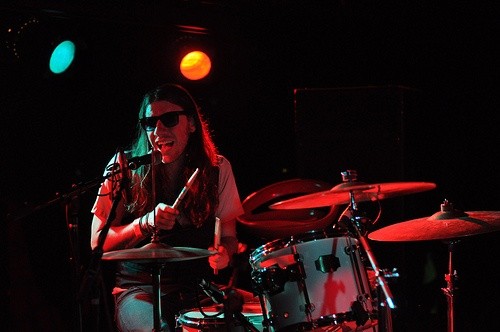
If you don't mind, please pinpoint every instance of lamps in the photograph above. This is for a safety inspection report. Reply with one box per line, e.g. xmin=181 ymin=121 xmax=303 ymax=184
xmin=165 ymin=24 xmax=218 ymax=82
xmin=3 ymin=24 xmax=77 ymax=75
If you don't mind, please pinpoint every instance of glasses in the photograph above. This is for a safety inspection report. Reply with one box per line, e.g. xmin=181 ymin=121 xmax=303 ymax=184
xmin=136 ymin=110 xmax=185 ymax=131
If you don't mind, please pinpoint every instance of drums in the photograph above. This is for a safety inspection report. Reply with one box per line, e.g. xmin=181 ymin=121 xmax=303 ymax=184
xmin=249 ymin=227 xmax=379 ymax=332
xmin=180 ymin=303 xmax=274 ymax=332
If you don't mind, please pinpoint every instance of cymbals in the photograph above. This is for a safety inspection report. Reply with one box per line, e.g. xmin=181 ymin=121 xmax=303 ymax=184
xmin=269 ymin=181 xmax=436 ymax=210
xmin=368 ymin=211 xmax=500 ymax=242
xmin=102 ymin=243 xmax=215 ymax=260
